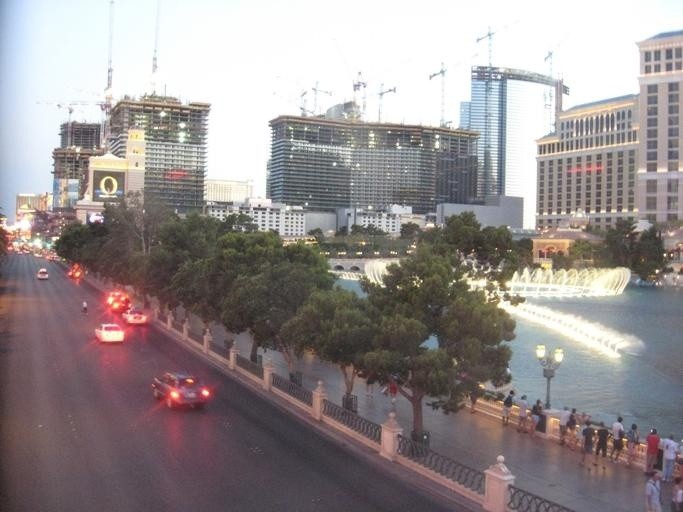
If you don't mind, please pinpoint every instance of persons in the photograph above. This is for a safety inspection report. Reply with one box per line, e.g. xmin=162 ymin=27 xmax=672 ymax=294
xmin=671 ymin=478 xmax=683 ymax=512
xmin=83 ymin=301 xmax=87 ymax=314
xmin=503 ymin=391 xmax=514 ymax=425
xmin=530 ymin=400 xmax=542 ymax=437
xmin=517 ymin=395 xmax=531 ymax=433
xmin=645 ymin=472 xmax=661 ymax=512
xmin=470 ymin=393 xmax=477 ymax=413
xmin=558 ymin=407 xmax=683 ymax=483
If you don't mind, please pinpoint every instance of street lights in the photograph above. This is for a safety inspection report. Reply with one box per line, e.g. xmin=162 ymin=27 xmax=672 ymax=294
xmin=535 ymin=343 xmax=564 ymax=409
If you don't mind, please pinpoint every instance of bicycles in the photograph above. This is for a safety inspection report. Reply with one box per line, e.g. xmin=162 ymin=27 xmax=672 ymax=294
xmin=80 ymin=305 xmax=88 ymax=317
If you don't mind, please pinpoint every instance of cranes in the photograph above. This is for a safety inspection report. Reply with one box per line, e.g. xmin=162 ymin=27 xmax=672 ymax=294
xmin=539 ymin=46 xmax=557 ymax=78
xmin=286 ymin=24 xmax=498 ymax=129
xmin=31 ymin=98 xmax=105 ymax=122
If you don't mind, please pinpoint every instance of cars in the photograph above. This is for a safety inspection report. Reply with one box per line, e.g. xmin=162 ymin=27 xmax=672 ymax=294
xmin=150 ymin=371 xmax=208 ymax=409
xmin=111 ymin=297 xmax=130 ymax=312
xmin=68 ymin=267 xmax=81 ymax=280
xmin=6 ymin=238 xmax=66 ymax=263
xmin=37 ymin=268 xmax=48 ymax=280
xmin=103 ymin=290 xmax=122 ymax=306
xmin=121 ymin=307 xmax=146 ymax=325
xmin=94 ymin=323 xmax=125 ymax=345
